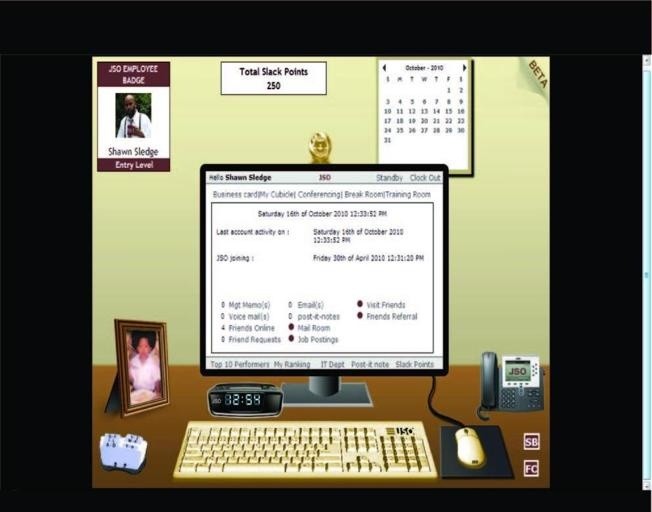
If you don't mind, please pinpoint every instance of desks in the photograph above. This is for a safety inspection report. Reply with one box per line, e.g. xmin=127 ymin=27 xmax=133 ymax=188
xmin=92 ymin=363 xmax=551 ymax=488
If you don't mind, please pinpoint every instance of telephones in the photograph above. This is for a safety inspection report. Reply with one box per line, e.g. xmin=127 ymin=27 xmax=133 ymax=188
xmin=481 ymin=352 xmax=545 ymax=412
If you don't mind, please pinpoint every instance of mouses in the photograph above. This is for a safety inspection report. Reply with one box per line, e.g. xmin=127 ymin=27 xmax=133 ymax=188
xmin=451 ymin=425 xmax=488 ymax=469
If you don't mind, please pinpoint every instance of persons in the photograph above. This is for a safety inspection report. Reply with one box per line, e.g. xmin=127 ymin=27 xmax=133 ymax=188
xmin=122 ymin=329 xmax=163 ymax=398
xmin=116 ymin=93 xmax=152 ymax=138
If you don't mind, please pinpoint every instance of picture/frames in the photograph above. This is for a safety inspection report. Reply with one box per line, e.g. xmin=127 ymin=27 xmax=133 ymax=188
xmin=114 ymin=318 xmax=172 ymax=419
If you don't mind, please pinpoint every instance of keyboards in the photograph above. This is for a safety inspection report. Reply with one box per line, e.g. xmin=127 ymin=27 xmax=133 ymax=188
xmin=172 ymin=419 xmax=435 ymax=476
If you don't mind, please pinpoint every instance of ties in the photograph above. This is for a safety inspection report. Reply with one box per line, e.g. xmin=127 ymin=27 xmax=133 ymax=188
xmin=127 ymin=120 xmax=133 ymax=138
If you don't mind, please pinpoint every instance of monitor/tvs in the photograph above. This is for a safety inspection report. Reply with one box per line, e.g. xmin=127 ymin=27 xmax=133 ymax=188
xmin=196 ymin=161 xmax=451 ymax=406
